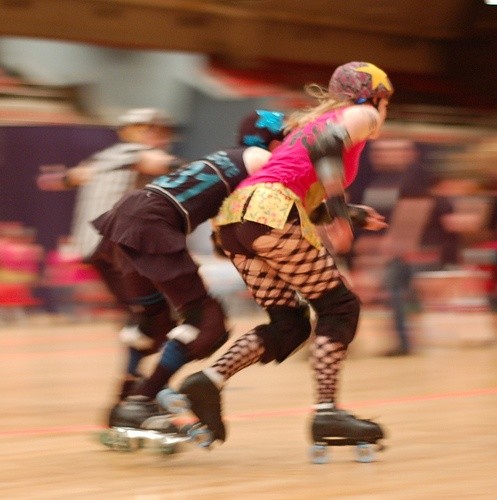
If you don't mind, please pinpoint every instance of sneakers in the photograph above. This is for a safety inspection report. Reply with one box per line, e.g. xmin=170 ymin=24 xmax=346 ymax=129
xmin=157 ymin=371 xmax=228 ymax=450
xmin=310 ymin=407 xmax=385 ymax=464
xmin=100 ymin=396 xmax=191 ymax=454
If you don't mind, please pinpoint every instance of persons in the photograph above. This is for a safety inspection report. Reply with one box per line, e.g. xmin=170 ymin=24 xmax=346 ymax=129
xmin=0 ymin=110 xmax=497 ymax=358
xmin=154 ymin=62 xmax=393 ymax=464
xmin=90 ymin=110 xmax=287 ymax=455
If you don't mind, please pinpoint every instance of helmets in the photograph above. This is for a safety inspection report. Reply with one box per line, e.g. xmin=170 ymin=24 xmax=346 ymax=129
xmin=329 ymin=61 xmax=394 ymax=104
xmin=237 ymin=109 xmax=287 ymax=150
